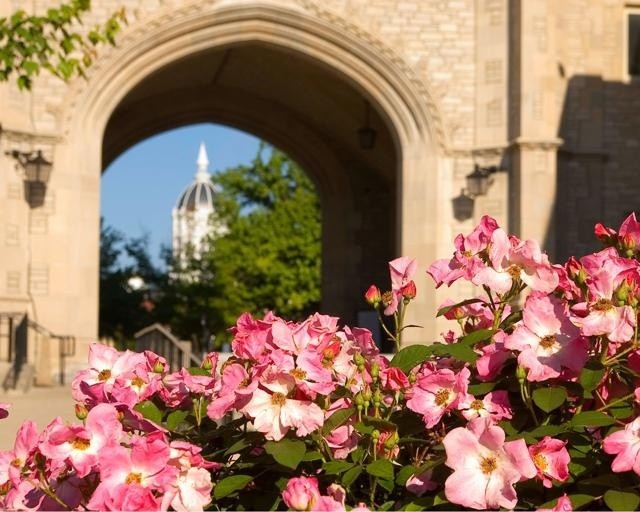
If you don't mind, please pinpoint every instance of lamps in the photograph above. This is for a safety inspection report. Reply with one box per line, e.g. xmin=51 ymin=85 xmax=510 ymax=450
xmin=467 ymin=158 xmax=509 ymax=196
xmin=5 ymin=148 xmax=53 ymax=186
